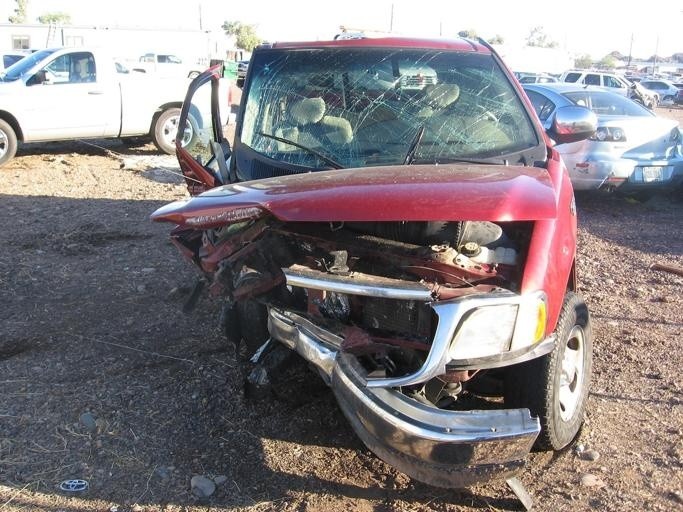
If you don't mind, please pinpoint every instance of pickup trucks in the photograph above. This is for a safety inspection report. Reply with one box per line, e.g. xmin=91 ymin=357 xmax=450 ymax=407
xmin=0 ymin=46 xmax=233 ymax=166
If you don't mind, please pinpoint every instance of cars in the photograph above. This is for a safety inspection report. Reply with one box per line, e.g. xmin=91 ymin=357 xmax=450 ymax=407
xmin=403 ymin=63 xmax=682 ymax=116
xmin=149 ymin=35 xmax=593 ymax=489
xmin=514 ymin=83 xmax=682 ymax=203
xmin=0 ymin=46 xmax=253 ymax=90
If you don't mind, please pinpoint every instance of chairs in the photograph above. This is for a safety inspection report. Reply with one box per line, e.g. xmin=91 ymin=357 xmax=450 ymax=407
xmin=417 ymin=83 xmax=460 ymax=120
xmin=276 ymin=98 xmax=354 ymax=154
xmin=69 ymin=60 xmax=80 ymax=84
xmin=83 ymin=61 xmax=96 ymax=84
